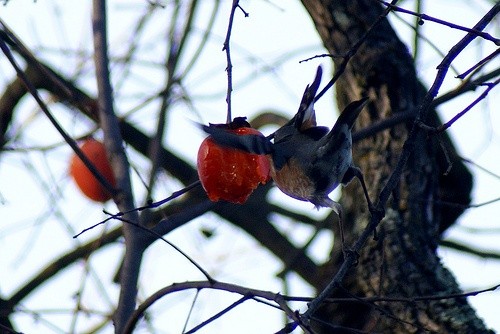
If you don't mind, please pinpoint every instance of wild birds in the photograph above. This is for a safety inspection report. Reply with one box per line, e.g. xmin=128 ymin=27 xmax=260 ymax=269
xmin=191 ymin=64 xmax=385 ymax=267
